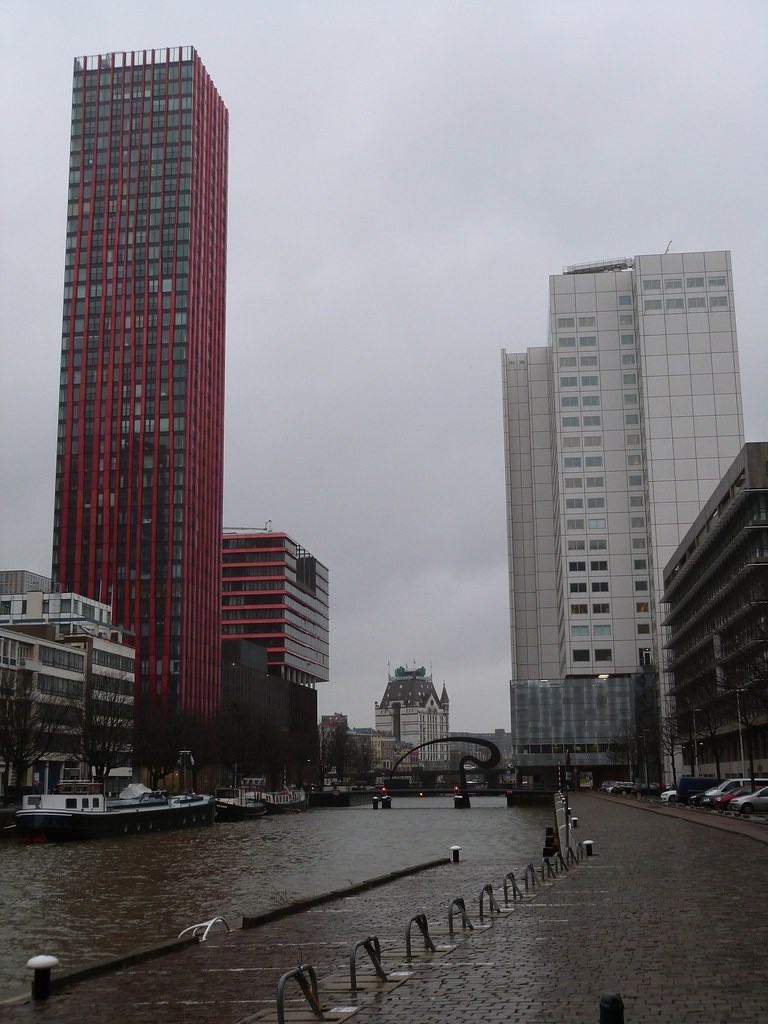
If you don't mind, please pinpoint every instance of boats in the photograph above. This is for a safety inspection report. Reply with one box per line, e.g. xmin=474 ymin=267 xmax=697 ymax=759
xmin=238 ymin=777 xmax=310 ymax=815
xmin=15 ymin=746 xmax=215 ymax=844
xmin=216 ymin=763 xmax=267 ymax=821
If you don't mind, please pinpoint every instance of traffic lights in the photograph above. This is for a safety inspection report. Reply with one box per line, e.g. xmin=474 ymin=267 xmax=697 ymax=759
xmin=382 ymin=787 xmax=386 ymax=795
xmin=454 ymin=787 xmax=459 ymax=794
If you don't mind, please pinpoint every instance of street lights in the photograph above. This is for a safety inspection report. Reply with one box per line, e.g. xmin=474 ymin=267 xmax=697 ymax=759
xmin=736 ymin=688 xmax=748 ymax=779
xmin=693 ymin=709 xmax=702 ymax=777
xmin=643 ymin=729 xmax=650 ymax=784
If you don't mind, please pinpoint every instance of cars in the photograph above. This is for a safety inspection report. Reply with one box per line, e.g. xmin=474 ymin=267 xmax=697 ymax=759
xmin=730 ymin=787 xmax=768 ymax=814
xmin=688 ymin=786 xmax=718 ymax=807
xmin=607 ymin=782 xmax=663 ymax=797
xmin=661 ymin=790 xmax=677 ymax=803
xmin=713 ymin=786 xmax=764 ymax=811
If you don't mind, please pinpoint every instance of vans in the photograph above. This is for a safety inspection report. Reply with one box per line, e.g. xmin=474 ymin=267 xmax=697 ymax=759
xmin=702 ymin=779 xmax=768 ymax=810
xmin=676 ymin=777 xmax=727 ymax=806
